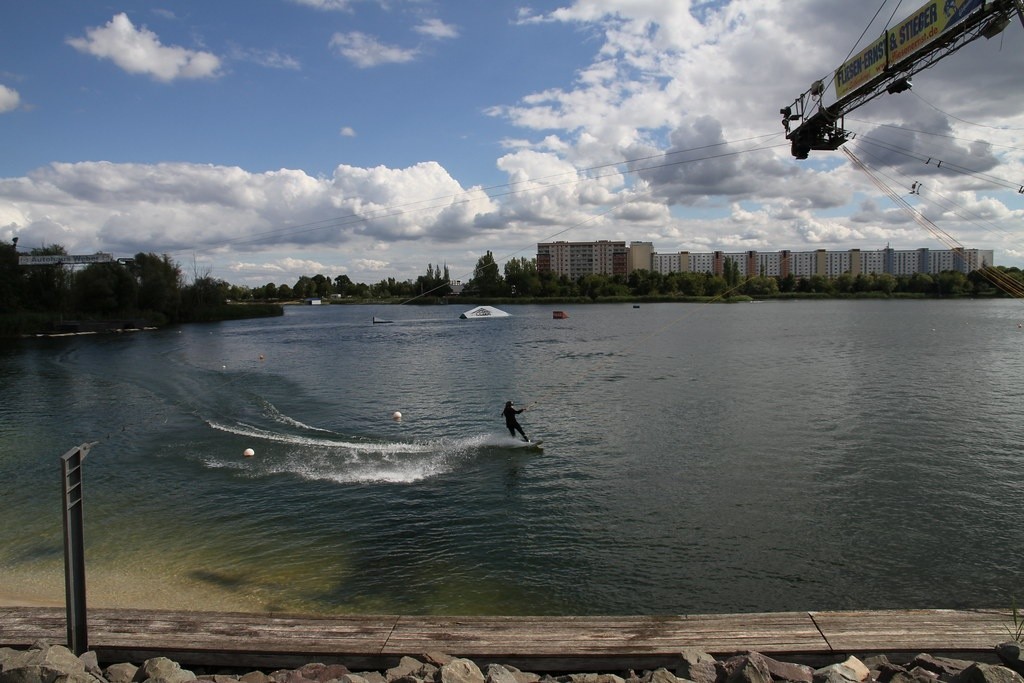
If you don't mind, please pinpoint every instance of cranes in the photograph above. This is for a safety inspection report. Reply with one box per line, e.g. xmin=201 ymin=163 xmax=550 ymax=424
xmin=779 ymin=1 xmax=1024 ymax=160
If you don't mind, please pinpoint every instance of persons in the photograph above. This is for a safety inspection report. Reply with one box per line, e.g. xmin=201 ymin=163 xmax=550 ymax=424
xmin=500 ymin=400 xmax=529 ymax=442
